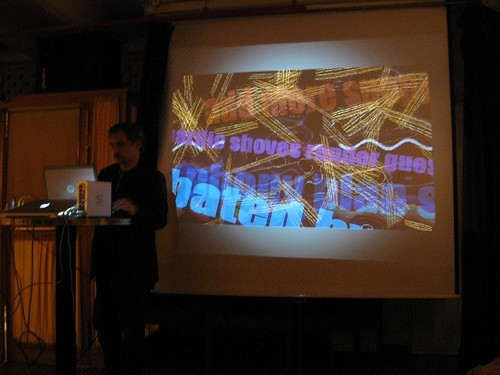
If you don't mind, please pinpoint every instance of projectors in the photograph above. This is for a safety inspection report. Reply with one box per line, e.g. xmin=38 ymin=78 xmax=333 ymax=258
xmin=76 ymin=178 xmax=111 ymax=218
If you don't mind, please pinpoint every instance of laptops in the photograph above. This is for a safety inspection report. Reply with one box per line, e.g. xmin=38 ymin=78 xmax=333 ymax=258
xmin=4 ymin=199 xmax=78 ymax=217
xmin=44 ymin=164 xmax=97 ymax=203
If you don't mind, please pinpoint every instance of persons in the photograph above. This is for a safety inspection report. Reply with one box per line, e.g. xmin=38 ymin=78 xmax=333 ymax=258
xmin=92 ymin=120 xmax=171 ymax=375
